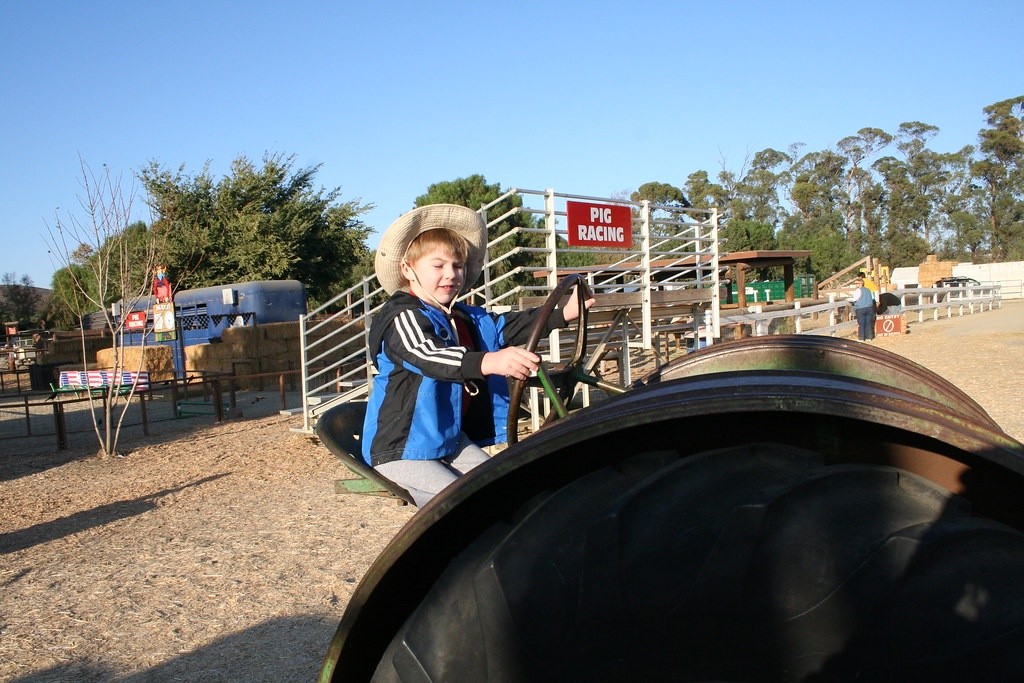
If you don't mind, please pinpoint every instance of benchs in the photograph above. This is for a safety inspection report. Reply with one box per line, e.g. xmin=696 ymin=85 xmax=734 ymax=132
xmin=50 ymin=370 xmax=152 ymax=402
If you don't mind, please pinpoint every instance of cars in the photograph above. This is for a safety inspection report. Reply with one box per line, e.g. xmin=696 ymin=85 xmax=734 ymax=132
xmin=930 ymin=276 xmax=996 ymax=303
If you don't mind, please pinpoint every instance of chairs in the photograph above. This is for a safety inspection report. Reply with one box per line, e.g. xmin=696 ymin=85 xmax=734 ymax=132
xmin=314 ymin=402 xmax=417 ymax=508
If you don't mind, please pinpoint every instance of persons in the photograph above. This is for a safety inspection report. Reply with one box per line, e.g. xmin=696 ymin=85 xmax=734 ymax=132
xmin=361 ymin=203 xmax=595 ymax=511
xmin=846 ymin=272 xmax=880 ymax=343
xmin=32 ymin=320 xmax=51 ymax=350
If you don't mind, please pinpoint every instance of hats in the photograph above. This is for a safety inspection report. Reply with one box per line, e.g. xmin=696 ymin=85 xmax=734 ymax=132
xmin=374 ymin=203 xmax=488 ymax=298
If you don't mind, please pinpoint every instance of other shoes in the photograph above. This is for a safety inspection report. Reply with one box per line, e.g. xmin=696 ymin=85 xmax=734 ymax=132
xmin=856 ymin=340 xmax=864 ymax=342
xmin=866 ymin=338 xmax=872 ymax=342
